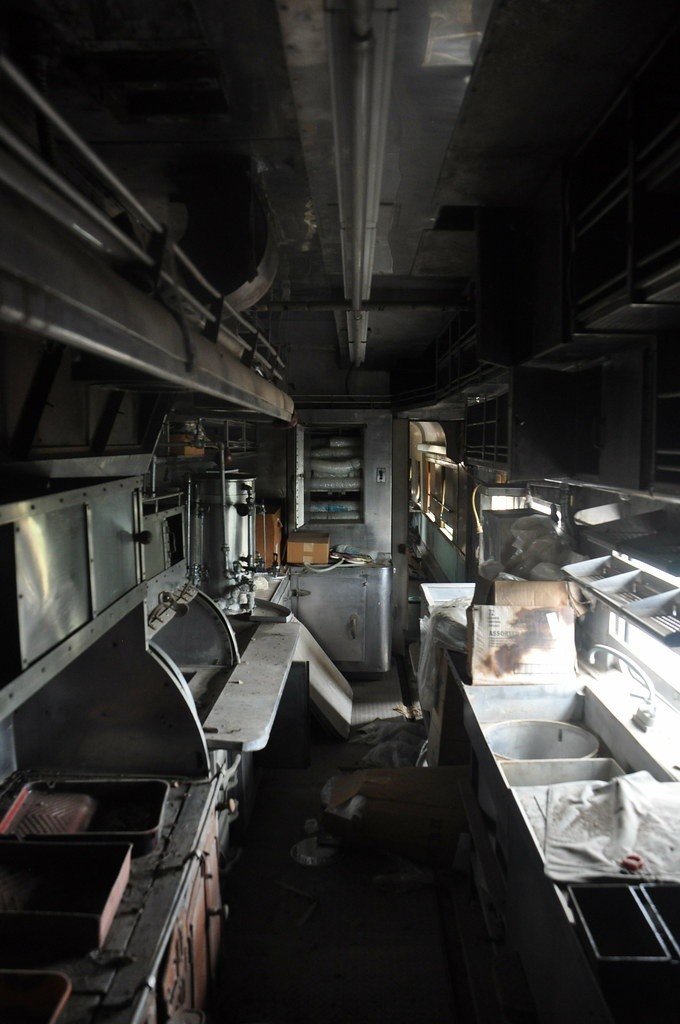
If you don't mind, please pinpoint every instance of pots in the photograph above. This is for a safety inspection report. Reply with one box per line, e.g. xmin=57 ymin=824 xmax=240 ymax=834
xmin=185 ymin=468 xmax=260 ymax=600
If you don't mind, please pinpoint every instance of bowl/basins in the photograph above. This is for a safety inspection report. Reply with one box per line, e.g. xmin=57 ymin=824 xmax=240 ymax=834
xmin=484 ymin=717 xmax=602 ymax=764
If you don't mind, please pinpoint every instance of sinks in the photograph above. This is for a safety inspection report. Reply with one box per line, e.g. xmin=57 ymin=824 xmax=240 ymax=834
xmin=462 ymin=682 xmax=679 ymax=792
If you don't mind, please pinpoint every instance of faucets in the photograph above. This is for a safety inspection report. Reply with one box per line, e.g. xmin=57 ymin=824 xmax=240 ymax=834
xmin=586 ymin=643 xmax=665 ymax=732
